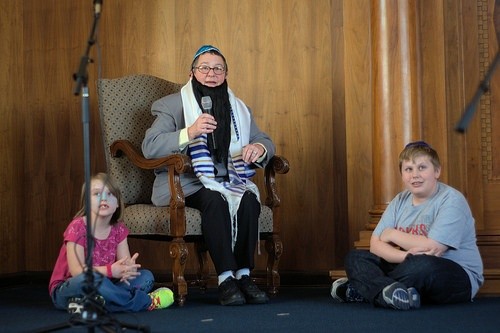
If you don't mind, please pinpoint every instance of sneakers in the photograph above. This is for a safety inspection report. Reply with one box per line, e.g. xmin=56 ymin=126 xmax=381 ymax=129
xmin=329 ymin=277 xmax=365 ymax=302
xmin=147 ymin=287 xmax=175 ymax=311
xmin=380 ymin=282 xmax=421 ymax=310
xmin=67 ymin=295 xmax=105 ymax=315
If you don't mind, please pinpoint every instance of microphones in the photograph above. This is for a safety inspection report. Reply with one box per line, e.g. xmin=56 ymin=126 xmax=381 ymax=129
xmin=93 ymin=0 xmax=102 ymax=18
xmin=202 ymin=96 xmax=218 ymax=150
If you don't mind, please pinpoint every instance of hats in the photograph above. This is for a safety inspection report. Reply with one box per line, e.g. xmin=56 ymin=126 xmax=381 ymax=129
xmin=405 ymin=141 xmax=429 ymax=148
xmin=192 ymin=44 xmax=221 ymax=61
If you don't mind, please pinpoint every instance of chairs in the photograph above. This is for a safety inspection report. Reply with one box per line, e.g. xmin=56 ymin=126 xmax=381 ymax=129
xmin=97 ymin=74 xmax=291 ymax=297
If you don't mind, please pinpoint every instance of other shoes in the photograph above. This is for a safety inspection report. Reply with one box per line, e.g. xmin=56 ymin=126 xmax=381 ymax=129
xmin=237 ymin=275 xmax=269 ymax=304
xmin=215 ymin=276 xmax=246 ymax=305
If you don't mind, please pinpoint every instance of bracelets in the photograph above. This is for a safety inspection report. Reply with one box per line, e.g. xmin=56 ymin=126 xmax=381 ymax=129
xmin=107 ymin=265 xmax=112 ymax=278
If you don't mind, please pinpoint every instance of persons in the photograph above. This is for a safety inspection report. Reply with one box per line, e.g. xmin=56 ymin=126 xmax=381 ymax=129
xmin=141 ymin=45 xmax=275 ymax=306
xmin=331 ymin=141 xmax=484 ymax=310
xmin=49 ymin=173 xmax=175 ymax=315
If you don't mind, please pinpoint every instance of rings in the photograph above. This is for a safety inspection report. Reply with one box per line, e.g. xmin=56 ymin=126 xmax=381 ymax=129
xmin=253 ymin=151 xmax=257 ymax=154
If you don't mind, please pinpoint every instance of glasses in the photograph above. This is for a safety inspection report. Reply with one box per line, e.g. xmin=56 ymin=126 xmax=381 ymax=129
xmin=193 ymin=64 xmax=226 ymax=75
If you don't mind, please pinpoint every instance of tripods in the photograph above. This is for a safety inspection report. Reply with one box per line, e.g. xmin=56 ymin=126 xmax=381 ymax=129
xmin=36 ymin=13 xmax=151 ymax=333
